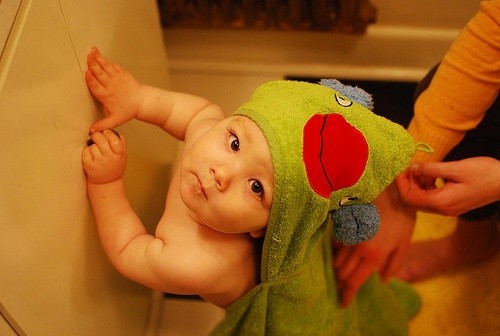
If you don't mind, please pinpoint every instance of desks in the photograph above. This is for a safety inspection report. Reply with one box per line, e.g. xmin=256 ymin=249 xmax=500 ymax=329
xmin=162 ymin=27 xmax=455 ymax=112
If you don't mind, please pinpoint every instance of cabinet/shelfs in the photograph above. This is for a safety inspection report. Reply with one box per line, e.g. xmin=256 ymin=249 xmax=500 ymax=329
xmin=3 ymin=0 xmax=184 ymax=336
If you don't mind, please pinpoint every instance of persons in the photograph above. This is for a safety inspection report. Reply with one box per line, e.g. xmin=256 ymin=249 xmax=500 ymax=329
xmin=82 ymin=47 xmax=423 ymax=335
xmin=331 ymin=0 xmax=500 ymax=310
